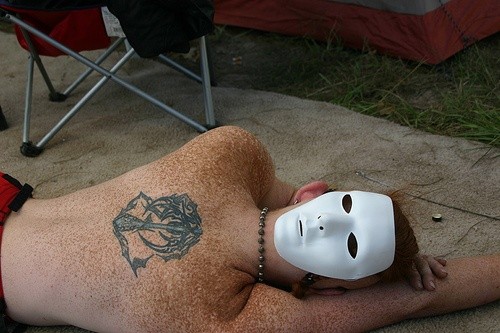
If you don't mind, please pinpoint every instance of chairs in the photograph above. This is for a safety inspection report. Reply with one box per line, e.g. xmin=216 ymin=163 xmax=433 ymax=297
xmin=0 ymin=0 xmax=217 ymax=158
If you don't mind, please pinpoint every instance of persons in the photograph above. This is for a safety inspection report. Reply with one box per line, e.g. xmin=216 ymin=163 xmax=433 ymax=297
xmin=0 ymin=126 xmax=500 ymax=333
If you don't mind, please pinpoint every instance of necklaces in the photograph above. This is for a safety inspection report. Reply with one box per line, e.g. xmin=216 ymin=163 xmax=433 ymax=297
xmin=258 ymin=207 xmax=269 ymax=283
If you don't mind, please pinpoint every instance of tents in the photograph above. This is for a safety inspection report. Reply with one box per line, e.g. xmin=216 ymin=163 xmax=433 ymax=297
xmin=213 ymin=0 xmax=500 ymax=68
